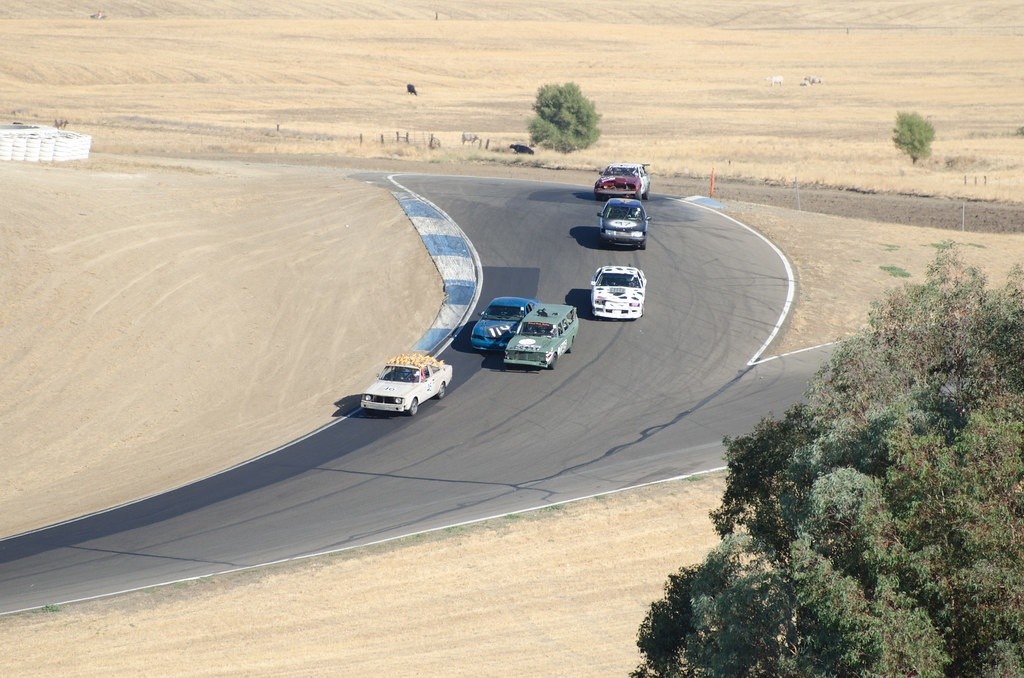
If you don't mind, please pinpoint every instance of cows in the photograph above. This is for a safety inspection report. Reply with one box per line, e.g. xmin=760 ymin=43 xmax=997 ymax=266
xmin=407 ymin=83 xmax=417 ymax=96
xmin=509 ymin=143 xmax=535 ymax=155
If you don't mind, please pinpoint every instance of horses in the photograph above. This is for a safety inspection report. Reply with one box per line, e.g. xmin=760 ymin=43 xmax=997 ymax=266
xmin=798 ymin=74 xmax=823 ymax=88
xmin=764 ymin=75 xmax=784 ymax=87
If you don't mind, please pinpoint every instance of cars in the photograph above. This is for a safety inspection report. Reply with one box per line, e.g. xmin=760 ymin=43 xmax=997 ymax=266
xmin=589 ymin=265 xmax=647 ymax=321
xmin=594 ymin=163 xmax=652 ymax=201
xmin=359 ymin=354 xmax=453 ymax=416
xmin=470 ymin=296 xmax=539 ymax=356
xmin=596 ymin=197 xmax=652 ymax=250
xmin=503 ymin=303 xmax=580 ymax=370
